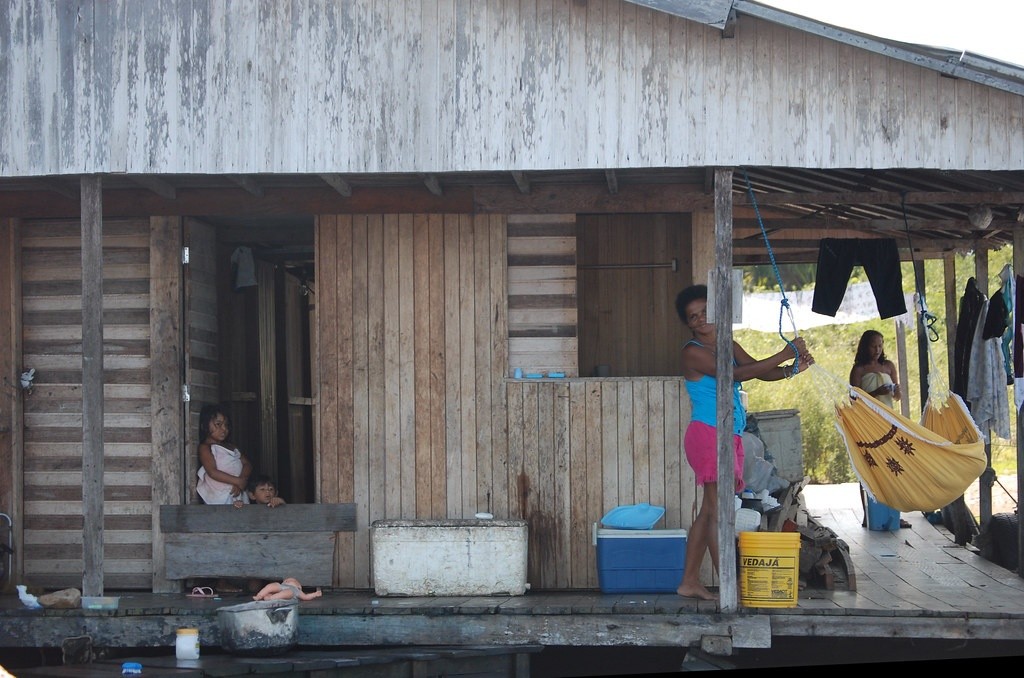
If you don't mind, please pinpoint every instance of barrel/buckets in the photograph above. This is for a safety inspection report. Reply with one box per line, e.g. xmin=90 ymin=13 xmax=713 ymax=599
xmin=862 ymin=488 xmax=900 ymax=531
xmin=738 ymin=531 xmax=802 ymax=608
xmin=747 ymin=409 xmax=804 ymax=481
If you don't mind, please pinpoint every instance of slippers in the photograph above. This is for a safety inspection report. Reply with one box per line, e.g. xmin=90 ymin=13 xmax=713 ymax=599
xmin=186 ymin=586 xmax=219 ymax=597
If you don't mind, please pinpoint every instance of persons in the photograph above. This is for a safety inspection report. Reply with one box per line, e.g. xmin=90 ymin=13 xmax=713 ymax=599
xmin=197 ymin=404 xmax=286 ymax=509
xmin=849 ymin=330 xmax=903 ymax=529
xmin=675 ymin=284 xmax=817 ymax=601
xmin=252 ymin=577 xmax=322 ymax=601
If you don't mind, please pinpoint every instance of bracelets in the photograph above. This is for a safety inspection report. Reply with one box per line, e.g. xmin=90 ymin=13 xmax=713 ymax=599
xmin=782 ymin=364 xmax=789 ymax=379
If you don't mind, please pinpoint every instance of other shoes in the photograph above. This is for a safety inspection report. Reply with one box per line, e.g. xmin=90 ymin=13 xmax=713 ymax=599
xmin=862 ymin=517 xmax=867 ymax=527
xmin=900 ymin=519 xmax=912 ymax=528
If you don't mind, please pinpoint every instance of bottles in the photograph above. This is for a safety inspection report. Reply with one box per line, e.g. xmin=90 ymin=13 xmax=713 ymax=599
xmin=176 ymin=629 xmax=200 ymax=660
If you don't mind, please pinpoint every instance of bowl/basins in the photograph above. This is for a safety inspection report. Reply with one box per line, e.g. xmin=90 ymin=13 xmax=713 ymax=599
xmin=81 ymin=597 xmax=121 ymax=610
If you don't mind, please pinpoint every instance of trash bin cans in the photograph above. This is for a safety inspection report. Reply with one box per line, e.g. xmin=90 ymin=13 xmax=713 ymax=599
xmin=866 ymin=491 xmax=901 ymax=532
